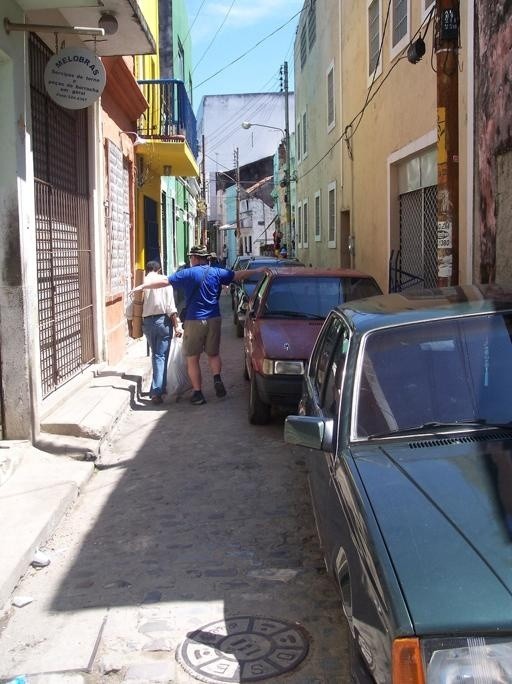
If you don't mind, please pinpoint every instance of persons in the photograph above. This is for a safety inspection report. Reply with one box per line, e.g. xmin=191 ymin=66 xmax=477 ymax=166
xmin=125 ymin=260 xmax=182 ymax=404
xmin=210 ymin=251 xmax=217 ymax=265
xmin=131 ymin=245 xmax=270 ymax=406
xmin=221 ymin=243 xmax=228 ymax=266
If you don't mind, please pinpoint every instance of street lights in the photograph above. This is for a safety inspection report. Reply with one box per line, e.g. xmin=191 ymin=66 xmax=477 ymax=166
xmin=179 ymin=267 xmax=211 ymax=321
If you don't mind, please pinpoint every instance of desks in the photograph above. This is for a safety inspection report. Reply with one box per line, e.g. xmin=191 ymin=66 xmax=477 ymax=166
xmin=242 ymin=118 xmax=294 ymax=258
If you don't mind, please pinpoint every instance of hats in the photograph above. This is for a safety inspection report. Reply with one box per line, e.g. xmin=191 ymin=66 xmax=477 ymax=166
xmin=244 ymin=267 xmax=385 ymax=424
xmin=230 ymin=256 xmax=306 ymax=337
xmin=283 ymin=283 xmax=512 ymax=684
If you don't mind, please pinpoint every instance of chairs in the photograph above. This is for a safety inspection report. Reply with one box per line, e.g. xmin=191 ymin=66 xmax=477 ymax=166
xmin=150 ymin=391 xmax=162 ymax=402
xmin=190 ymin=391 xmax=206 ymax=405
xmin=214 ymin=375 xmax=226 ymax=398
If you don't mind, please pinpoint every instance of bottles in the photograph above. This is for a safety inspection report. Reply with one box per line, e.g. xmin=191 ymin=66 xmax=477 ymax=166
xmin=188 ymin=245 xmax=212 ymax=257
xmin=210 ymin=252 xmax=217 ymax=259
